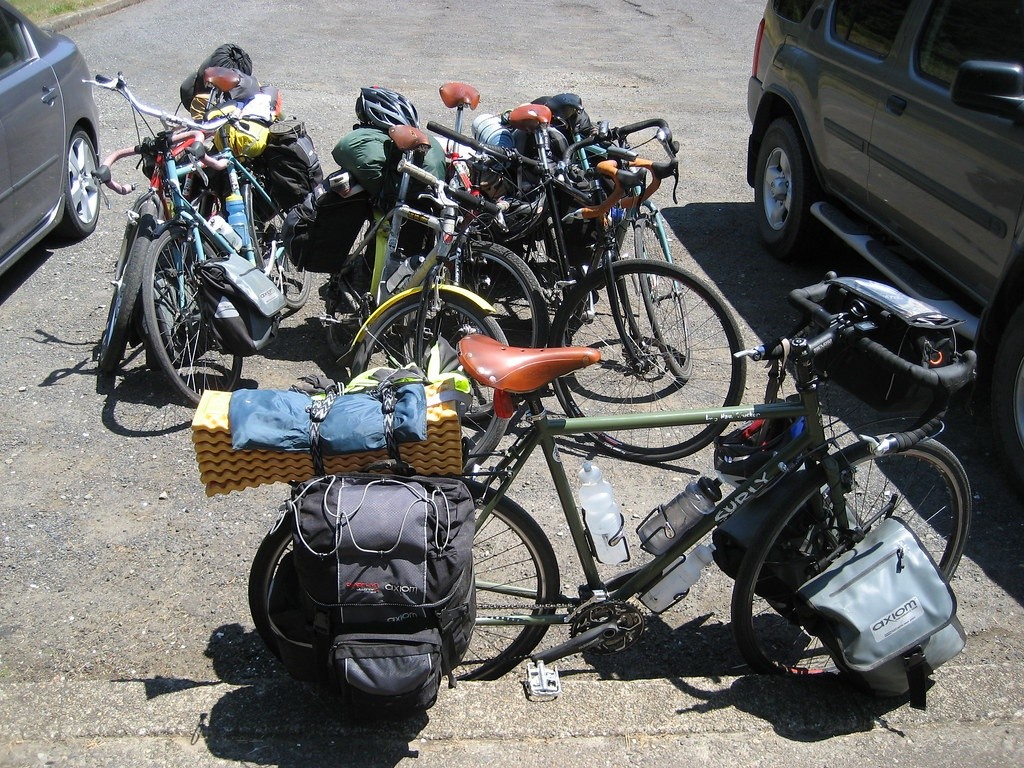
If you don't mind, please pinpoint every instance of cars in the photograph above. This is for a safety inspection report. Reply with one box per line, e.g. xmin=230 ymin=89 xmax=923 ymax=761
xmin=0 ymin=0 xmax=105 ymax=277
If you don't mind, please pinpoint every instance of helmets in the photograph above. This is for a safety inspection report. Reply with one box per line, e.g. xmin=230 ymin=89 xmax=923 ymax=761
xmin=358 ymin=84 xmax=421 ymax=129
xmin=710 ymin=395 xmax=808 ymax=487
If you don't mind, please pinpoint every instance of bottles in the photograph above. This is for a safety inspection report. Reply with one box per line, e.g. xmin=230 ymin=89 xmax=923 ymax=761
xmin=638 ymin=476 xmax=722 ymax=555
xmin=578 ymin=462 xmax=629 ymax=565
xmin=226 ymin=193 xmax=250 ymax=246
xmin=387 ymin=255 xmax=425 ymax=294
xmin=208 ymin=215 xmax=242 ymax=250
xmin=637 ymin=543 xmax=716 ymax=612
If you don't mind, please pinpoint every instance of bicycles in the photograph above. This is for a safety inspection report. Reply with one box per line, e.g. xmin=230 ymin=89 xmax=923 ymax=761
xmin=246 ymin=272 xmax=971 ymax=697
xmin=79 ymin=61 xmax=749 ymax=462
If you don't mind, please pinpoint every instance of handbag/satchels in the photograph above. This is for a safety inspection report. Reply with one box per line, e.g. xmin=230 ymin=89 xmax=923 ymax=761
xmin=281 ymin=166 xmax=371 ymax=273
xmin=193 ymin=253 xmax=288 ymax=355
xmin=290 ymin=476 xmax=478 ymax=719
xmin=792 ymin=516 xmax=968 ymax=710
xmin=260 ymin=115 xmax=323 ymax=214
xmin=713 ymin=471 xmax=862 ymax=627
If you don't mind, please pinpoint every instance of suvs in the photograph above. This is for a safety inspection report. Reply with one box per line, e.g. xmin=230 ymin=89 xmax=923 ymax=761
xmin=743 ymin=0 xmax=1024 ymax=502
